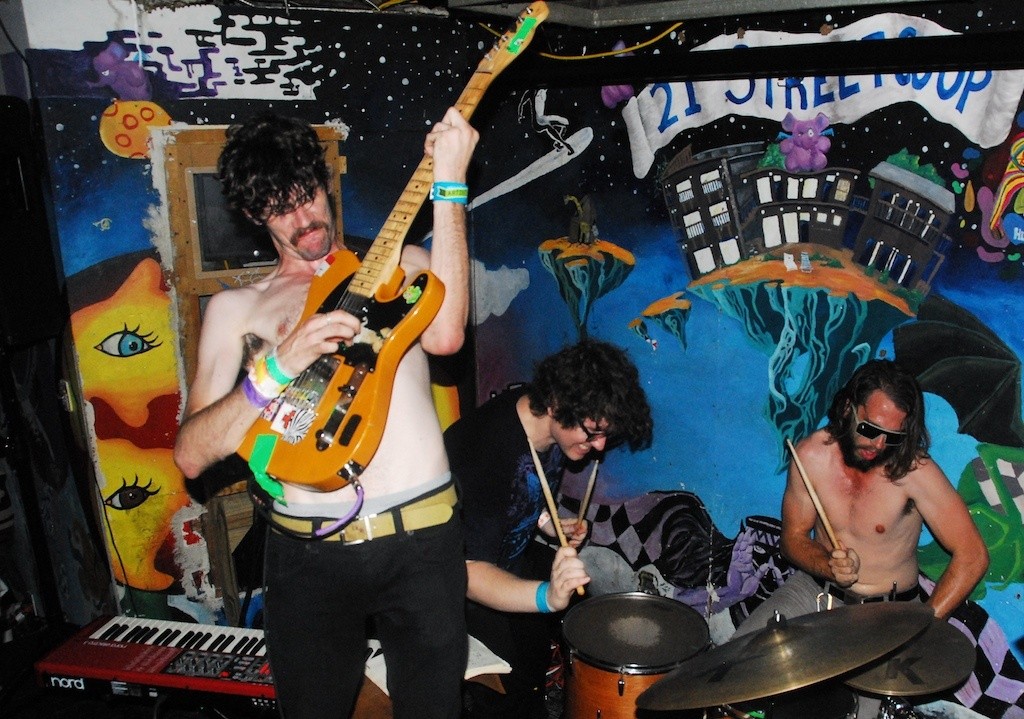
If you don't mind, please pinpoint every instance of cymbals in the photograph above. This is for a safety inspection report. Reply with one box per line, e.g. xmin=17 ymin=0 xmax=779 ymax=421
xmin=634 ymin=600 xmax=935 ymax=711
xmin=844 ymin=614 xmax=975 ymax=697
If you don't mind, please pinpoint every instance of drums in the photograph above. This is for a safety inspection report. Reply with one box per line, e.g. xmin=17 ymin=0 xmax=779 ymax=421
xmin=547 ymin=589 xmax=718 ymax=719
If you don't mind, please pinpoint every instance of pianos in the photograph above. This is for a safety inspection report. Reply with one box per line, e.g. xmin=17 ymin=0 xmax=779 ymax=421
xmin=33 ymin=612 xmax=279 ymax=719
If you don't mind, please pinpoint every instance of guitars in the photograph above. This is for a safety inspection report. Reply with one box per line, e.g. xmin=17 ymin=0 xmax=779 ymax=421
xmin=237 ymin=1 xmax=549 ymax=495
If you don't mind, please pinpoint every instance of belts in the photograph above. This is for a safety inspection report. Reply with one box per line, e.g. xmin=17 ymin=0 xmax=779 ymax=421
xmin=812 ymin=574 xmax=919 ymax=606
xmin=271 ymin=483 xmax=458 ymax=548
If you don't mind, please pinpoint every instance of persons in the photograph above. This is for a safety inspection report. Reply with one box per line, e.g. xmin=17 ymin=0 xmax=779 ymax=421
xmin=440 ymin=335 xmax=654 ymax=718
xmin=728 ymin=356 xmax=992 ymax=719
xmin=173 ymin=106 xmax=480 ymax=719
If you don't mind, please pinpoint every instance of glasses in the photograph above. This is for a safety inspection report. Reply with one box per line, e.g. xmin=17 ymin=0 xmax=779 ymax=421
xmin=850 ymin=403 xmax=908 ymax=446
xmin=579 ymin=421 xmax=616 ymax=442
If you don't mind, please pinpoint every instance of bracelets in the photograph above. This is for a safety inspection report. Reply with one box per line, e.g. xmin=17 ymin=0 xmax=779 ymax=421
xmin=429 ymin=181 xmax=469 ymax=205
xmin=537 ymin=508 xmax=552 ymax=528
xmin=241 ymin=347 xmax=300 ymax=408
xmin=535 ymin=581 xmax=554 ymax=613
